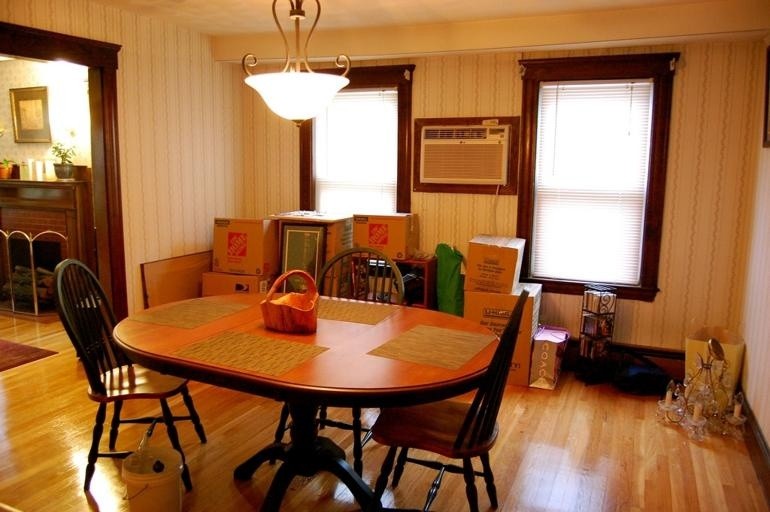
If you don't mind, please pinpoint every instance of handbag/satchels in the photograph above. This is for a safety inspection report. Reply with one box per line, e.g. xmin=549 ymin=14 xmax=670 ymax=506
xmin=529 ymin=323 xmax=570 ymax=391
xmin=683 ymin=324 xmax=745 ymax=412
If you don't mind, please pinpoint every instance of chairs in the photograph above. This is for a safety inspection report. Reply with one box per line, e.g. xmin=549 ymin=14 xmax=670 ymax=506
xmin=370 ymin=290 xmax=530 ymax=511
xmin=270 ymin=249 xmax=404 ymax=477
xmin=55 ymin=259 xmax=207 ymax=491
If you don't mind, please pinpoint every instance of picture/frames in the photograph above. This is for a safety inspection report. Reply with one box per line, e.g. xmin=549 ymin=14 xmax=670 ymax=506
xmin=9 ymin=87 xmax=53 ymax=144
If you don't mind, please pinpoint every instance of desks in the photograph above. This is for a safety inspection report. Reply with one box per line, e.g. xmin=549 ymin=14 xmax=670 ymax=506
xmin=112 ymin=291 xmax=503 ymax=512
xmin=0 ymin=179 xmax=96 ymax=315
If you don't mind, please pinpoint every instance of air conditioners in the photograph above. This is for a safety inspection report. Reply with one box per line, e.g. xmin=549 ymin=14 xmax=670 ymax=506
xmin=419 ymin=124 xmax=511 ymax=184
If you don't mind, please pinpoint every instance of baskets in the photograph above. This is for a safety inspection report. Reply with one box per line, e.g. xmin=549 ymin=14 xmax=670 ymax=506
xmin=260 ymin=270 xmax=319 ymax=335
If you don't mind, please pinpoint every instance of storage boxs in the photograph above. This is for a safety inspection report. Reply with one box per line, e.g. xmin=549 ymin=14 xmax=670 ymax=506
xmin=457 ymin=232 xmax=570 ymax=393
xmin=198 ymin=212 xmax=421 ymax=308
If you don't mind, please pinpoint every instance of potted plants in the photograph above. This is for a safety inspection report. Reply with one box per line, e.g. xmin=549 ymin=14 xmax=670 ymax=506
xmin=51 ymin=142 xmax=79 ymax=179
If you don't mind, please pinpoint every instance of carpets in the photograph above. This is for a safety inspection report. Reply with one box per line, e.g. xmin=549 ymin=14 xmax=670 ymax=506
xmin=0 ymin=338 xmax=58 ymax=372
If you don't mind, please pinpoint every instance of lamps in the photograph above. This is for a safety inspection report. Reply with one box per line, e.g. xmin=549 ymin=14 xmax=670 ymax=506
xmin=655 ymin=339 xmax=747 ymax=446
xmin=242 ymin=1 xmax=351 ymax=128
